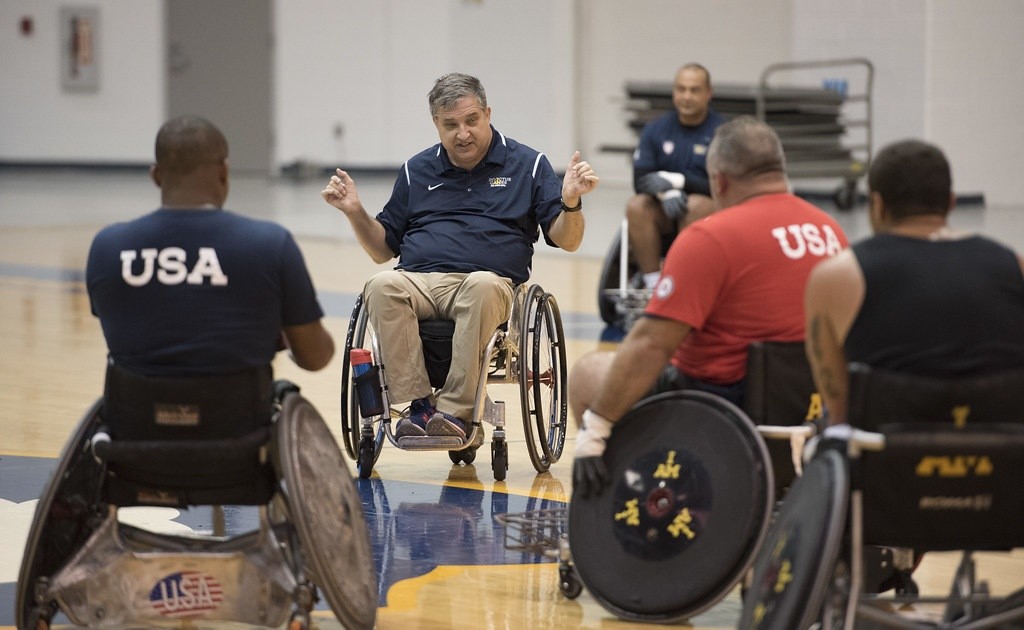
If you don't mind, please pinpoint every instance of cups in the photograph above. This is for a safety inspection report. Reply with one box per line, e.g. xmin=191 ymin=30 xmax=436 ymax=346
xmin=350 ymin=348 xmax=384 ymax=418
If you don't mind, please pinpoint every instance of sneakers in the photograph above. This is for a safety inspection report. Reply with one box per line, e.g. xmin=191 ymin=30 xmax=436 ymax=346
xmin=396 ymin=398 xmax=436 ymax=442
xmin=426 ymin=412 xmax=472 ymax=444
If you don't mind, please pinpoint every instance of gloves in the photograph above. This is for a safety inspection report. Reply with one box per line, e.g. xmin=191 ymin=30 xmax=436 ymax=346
xmin=639 ymin=170 xmax=685 ymax=193
xmin=573 ymin=409 xmax=615 ymax=498
xmin=655 ymin=189 xmax=687 ymax=219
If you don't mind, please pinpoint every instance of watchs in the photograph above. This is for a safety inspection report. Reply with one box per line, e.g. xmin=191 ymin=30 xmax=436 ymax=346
xmin=560 ymin=196 xmax=582 ymax=212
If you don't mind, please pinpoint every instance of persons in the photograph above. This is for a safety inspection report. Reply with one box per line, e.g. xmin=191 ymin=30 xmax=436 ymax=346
xmin=567 ymin=116 xmax=848 ymax=498
xmin=322 ymin=73 xmax=600 ymax=443
xmin=627 ymin=63 xmax=726 ymax=289
xmin=85 ymin=114 xmax=335 ymax=372
xmin=805 ymin=141 xmax=1024 ymax=427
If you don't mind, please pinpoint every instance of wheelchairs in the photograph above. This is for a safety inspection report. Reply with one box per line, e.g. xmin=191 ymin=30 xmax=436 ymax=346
xmin=16 ymin=353 xmax=378 ymax=630
xmin=341 ymin=284 xmax=568 ymax=480
xmin=559 ymin=217 xmax=1024 ymax=630
